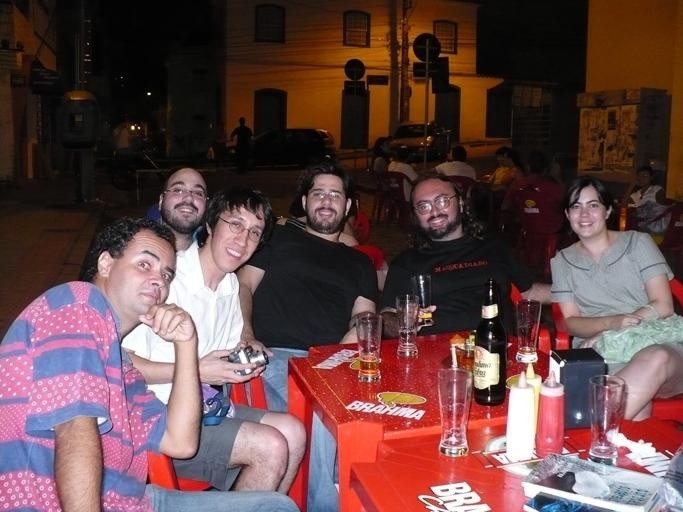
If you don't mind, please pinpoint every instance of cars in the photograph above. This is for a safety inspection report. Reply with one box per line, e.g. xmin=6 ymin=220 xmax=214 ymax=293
xmin=225 ymin=127 xmax=338 ymax=171
xmin=388 ymin=121 xmax=450 ymax=163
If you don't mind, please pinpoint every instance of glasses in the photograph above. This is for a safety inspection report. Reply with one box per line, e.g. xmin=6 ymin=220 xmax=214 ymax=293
xmin=309 ymin=192 xmax=346 ymax=205
xmin=413 ymin=194 xmax=459 ymax=215
xmin=218 ymin=216 xmax=263 ymax=244
xmin=165 ymin=188 xmax=209 ymax=203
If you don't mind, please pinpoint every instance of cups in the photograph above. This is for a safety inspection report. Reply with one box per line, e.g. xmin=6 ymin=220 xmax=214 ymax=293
xmin=435 ymin=368 xmax=474 ymax=459
xmin=394 ymin=294 xmax=421 ymax=358
xmin=408 ymin=275 xmax=435 ymax=327
xmin=586 ymin=373 xmax=626 ymax=460
xmin=515 ymin=298 xmax=541 ymax=364
xmin=355 ymin=312 xmax=385 ymax=383
xmin=453 ymin=343 xmax=475 ymax=372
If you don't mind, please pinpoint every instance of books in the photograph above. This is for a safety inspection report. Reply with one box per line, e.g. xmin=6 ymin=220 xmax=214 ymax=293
xmin=521 ymin=453 xmax=663 ymax=512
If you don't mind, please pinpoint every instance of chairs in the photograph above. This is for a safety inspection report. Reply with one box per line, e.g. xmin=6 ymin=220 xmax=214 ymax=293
xmin=441 ymin=175 xmax=475 ymax=215
xmin=370 ymin=171 xmax=417 ymax=227
xmin=510 ymin=188 xmax=562 ymax=284
xmin=658 ymin=206 xmax=683 ymax=273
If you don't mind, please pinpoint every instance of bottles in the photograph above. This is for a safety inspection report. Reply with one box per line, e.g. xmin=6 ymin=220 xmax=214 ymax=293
xmin=473 ymin=277 xmax=509 ymax=407
xmin=522 ymin=362 xmax=542 ymax=436
xmin=506 ymin=371 xmax=537 ymax=463
xmin=534 ymin=370 xmax=565 ymax=459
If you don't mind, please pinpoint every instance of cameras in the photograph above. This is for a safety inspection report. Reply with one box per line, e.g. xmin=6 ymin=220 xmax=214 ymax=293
xmin=229 ymin=344 xmax=270 ymax=375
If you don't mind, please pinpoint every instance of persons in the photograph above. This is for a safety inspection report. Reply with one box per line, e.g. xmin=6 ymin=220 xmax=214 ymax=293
xmin=552 ymin=178 xmax=682 ymax=421
xmin=236 ymin=166 xmax=386 ymax=512
xmin=371 ymin=137 xmax=419 ymax=223
xmin=435 ymin=145 xmax=574 ymax=262
xmin=380 ymin=173 xmax=517 ymax=336
xmin=0 ymin=219 xmax=301 ymax=512
xmin=157 ymin=132 xmax=174 ymax=167
xmin=629 ymin=164 xmax=673 ymax=245
xmin=230 ymin=118 xmax=254 ymax=171
xmin=118 ymin=164 xmax=307 ymax=494
